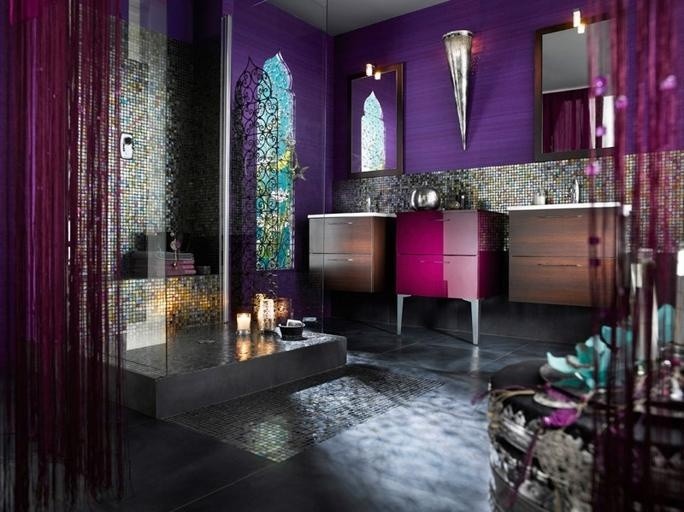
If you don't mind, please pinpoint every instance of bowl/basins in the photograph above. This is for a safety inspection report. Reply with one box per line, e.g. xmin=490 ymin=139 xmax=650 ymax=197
xmin=279 ymin=322 xmax=306 ymax=340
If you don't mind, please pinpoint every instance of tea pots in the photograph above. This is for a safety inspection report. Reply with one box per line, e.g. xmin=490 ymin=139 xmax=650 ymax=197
xmin=411 ymin=180 xmax=440 ymax=211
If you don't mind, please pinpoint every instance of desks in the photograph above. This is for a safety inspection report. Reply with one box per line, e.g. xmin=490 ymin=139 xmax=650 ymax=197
xmin=395 ymin=210 xmax=504 ymax=345
xmin=487 ymin=358 xmax=684 ymax=512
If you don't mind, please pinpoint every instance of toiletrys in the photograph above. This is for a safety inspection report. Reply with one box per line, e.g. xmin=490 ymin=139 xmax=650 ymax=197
xmin=533 ymin=189 xmax=546 ymax=205
xmin=458 ymin=182 xmax=467 ymax=208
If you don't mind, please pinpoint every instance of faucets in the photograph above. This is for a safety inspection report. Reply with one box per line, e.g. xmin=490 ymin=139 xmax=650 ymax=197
xmin=569 ymin=179 xmax=579 ymax=203
xmin=366 ymin=198 xmax=379 ymax=211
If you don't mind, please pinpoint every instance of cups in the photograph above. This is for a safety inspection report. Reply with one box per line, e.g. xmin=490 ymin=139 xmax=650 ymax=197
xmin=534 ymin=192 xmax=545 ymax=205
xmin=260 ymin=299 xmax=275 ymax=331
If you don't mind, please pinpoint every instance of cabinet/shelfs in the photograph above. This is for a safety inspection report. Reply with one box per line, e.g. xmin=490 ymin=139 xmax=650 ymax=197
xmin=508 ymin=207 xmax=622 ymax=310
xmin=309 ymin=218 xmax=393 ymax=294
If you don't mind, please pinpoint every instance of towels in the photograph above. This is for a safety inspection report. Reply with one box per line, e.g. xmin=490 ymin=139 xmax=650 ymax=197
xmin=132 ymin=251 xmax=197 ymax=276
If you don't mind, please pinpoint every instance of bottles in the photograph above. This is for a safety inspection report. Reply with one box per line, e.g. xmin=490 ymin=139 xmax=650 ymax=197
xmin=570 ymin=179 xmax=580 ymax=204
xmin=627 ymin=249 xmax=661 ymax=396
xmin=458 ymin=189 xmax=466 ymax=209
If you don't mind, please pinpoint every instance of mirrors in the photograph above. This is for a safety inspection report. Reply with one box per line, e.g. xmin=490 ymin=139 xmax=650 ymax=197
xmin=346 ymin=64 xmax=404 ymax=180
xmin=533 ymin=10 xmax=623 ymax=162
